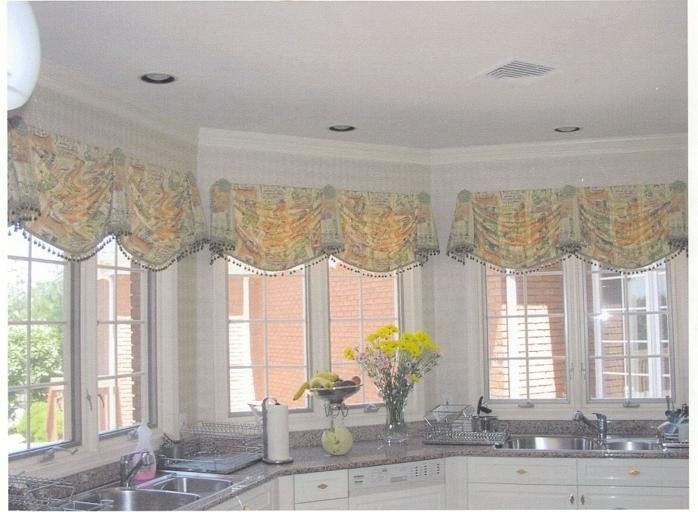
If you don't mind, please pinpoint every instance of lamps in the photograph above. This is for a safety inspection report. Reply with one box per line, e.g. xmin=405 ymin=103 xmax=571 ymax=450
xmin=7 ymin=1 xmax=41 ymax=115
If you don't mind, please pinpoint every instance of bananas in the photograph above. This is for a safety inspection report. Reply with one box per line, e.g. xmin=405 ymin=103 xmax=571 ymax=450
xmin=293 ymin=371 xmax=338 ymax=401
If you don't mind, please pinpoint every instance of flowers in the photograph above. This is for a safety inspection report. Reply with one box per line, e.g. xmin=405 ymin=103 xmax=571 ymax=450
xmin=343 ymin=325 xmax=442 ymax=435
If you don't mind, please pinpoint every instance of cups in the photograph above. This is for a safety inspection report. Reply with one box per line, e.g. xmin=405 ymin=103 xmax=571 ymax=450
xmin=474 ymin=416 xmax=496 ymax=433
xmin=162 ymin=444 xmax=183 ymax=463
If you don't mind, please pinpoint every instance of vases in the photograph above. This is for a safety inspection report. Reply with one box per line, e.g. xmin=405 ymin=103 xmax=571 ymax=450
xmin=378 ymin=406 xmax=411 ymax=447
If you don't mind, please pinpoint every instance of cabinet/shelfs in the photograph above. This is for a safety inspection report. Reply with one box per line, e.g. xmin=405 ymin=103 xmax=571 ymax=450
xmin=465 ymin=459 xmax=688 ymax=510
xmin=294 ymin=470 xmax=346 ymax=510
xmin=347 ymin=459 xmax=444 ymax=510
xmin=208 ymin=480 xmax=279 ymax=511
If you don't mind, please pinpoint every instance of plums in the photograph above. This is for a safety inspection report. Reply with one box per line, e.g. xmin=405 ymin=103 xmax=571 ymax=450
xmin=342 ymin=380 xmax=355 ymax=386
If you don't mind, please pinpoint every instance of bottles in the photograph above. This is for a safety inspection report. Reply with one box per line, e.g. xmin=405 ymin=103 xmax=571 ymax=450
xmin=677 ymin=405 xmax=689 ymax=444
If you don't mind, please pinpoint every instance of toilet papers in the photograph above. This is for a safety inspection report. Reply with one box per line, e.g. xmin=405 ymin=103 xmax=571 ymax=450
xmin=267 ymin=405 xmax=290 ymax=461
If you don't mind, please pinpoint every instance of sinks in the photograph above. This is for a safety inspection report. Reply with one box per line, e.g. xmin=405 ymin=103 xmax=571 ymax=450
xmin=155 ymin=477 xmax=233 ymax=499
xmin=605 ymin=439 xmax=666 ymax=453
xmin=495 ymin=435 xmax=604 ymax=454
xmin=58 ymin=491 xmax=199 ymax=511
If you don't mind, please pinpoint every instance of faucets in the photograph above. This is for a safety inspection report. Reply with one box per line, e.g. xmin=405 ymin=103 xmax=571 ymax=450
xmin=573 ymin=409 xmax=608 ymax=437
xmin=120 ymin=453 xmax=153 ymax=486
